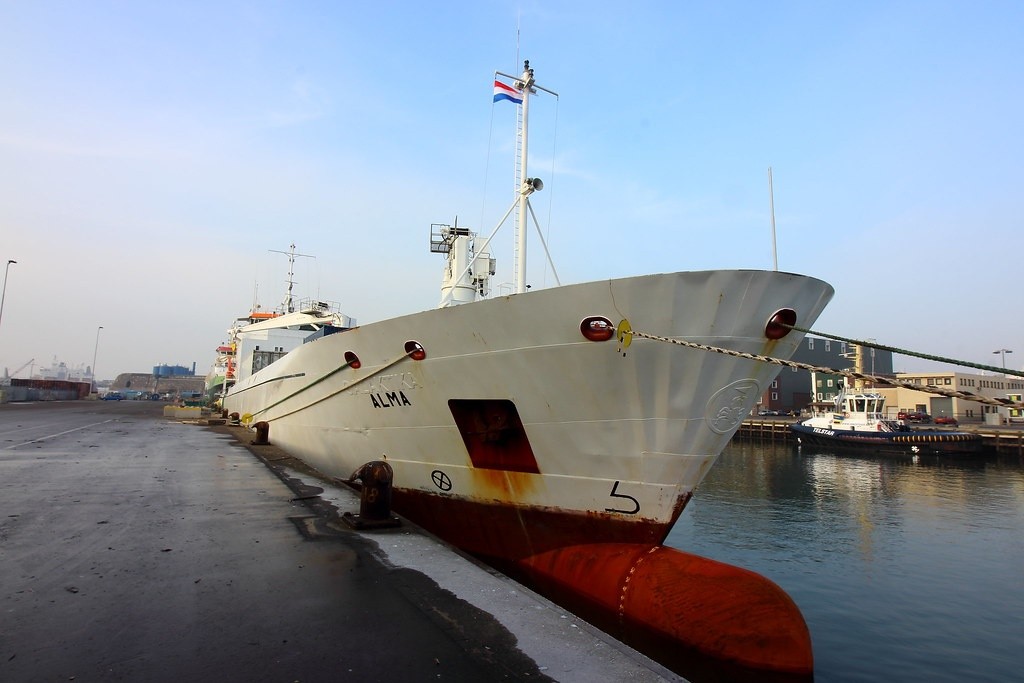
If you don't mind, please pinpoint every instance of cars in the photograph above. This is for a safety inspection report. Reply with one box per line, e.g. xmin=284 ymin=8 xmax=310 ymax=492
xmin=757 ymin=409 xmax=799 ymax=416
xmin=898 ymin=413 xmax=958 ymax=425
xmin=100 ymin=394 xmax=123 ymax=401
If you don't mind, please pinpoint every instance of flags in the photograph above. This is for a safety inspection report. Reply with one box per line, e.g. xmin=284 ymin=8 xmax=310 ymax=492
xmin=492 ymin=79 xmax=525 ymax=107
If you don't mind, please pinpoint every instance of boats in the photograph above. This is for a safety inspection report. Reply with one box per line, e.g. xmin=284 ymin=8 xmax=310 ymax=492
xmin=788 ymin=348 xmax=983 ymax=457
xmin=204 ymin=29 xmax=836 ymax=683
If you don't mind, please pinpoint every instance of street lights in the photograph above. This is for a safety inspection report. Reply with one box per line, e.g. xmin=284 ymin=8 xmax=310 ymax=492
xmin=1 ymin=260 xmax=17 ymax=326
xmin=993 ymin=350 xmax=1012 ymax=399
xmin=89 ymin=327 xmax=104 ymax=393
